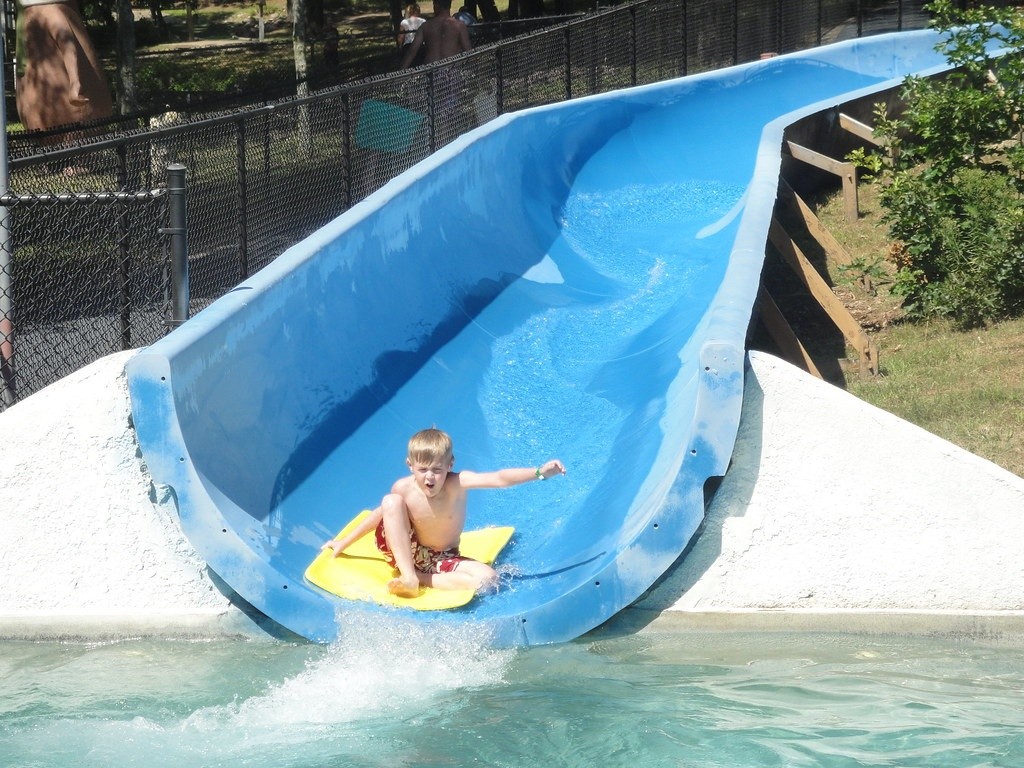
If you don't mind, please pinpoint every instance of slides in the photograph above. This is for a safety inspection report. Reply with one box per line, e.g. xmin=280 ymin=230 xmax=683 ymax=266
xmin=118 ymin=18 xmax=1023 ymax=652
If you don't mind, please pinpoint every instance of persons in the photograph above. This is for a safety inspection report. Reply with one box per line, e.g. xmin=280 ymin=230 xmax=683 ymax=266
xmin=399 ymin=0 xmax=481 ymax=136
xmin=322 ymin=429 xmax=567 ymax=599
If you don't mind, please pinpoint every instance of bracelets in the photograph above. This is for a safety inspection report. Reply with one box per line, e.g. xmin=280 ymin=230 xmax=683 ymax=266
xmin=535 ymin=468 xmax=546 ymax=481
